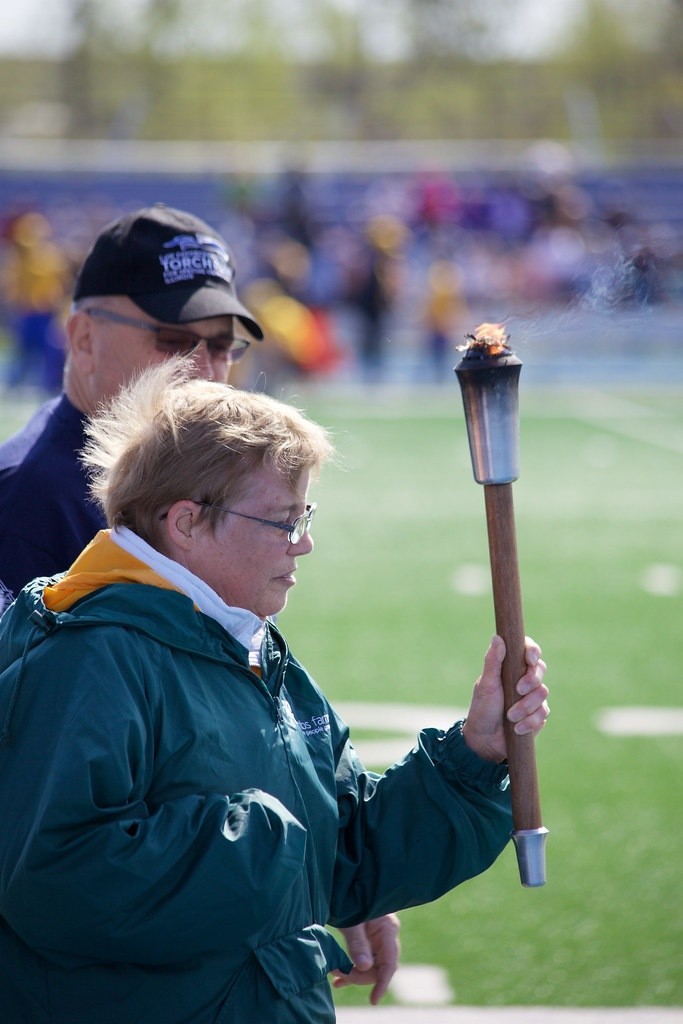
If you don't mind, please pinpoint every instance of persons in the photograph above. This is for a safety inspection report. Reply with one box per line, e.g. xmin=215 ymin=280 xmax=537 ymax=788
xmin=0 ymin=353 xmax=551 ymax=1024
xmin=0 ymin=201 xmax=398 ymax=1006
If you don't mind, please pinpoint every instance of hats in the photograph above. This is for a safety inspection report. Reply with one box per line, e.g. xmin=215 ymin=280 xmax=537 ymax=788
xmin=72 ymin=204 xmax=264 ymax=343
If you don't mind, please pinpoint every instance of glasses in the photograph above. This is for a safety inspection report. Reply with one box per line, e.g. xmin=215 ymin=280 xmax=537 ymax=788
xmin=82 ymin=307 xmax=251 ymax=364
xmin=159 ymin=501 xmax=317 ymax=544
